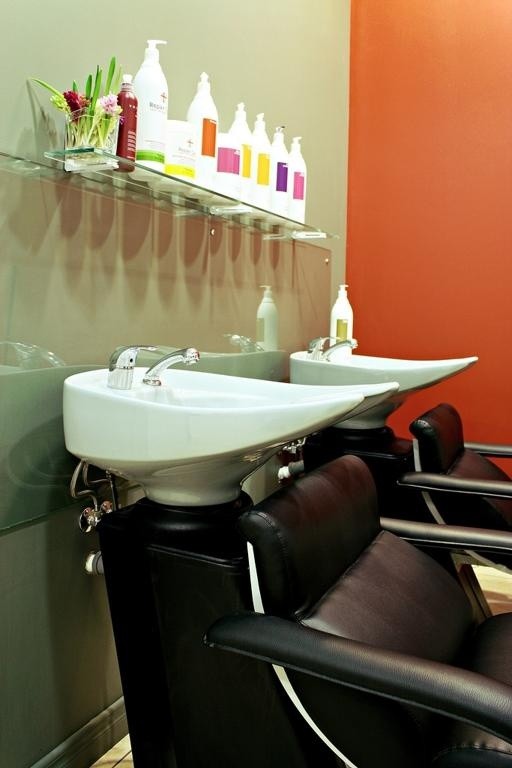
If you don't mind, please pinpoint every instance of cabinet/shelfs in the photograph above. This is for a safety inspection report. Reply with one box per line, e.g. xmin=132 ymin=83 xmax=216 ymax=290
xmin=43 ymin=147 xmax=340 ymax=241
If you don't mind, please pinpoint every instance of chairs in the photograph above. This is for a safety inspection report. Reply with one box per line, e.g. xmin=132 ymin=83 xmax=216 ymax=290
xmin=396 ymin=403 xmax=511 ymax=576
xmin=203 ymin=454 xmax=512 ymax=768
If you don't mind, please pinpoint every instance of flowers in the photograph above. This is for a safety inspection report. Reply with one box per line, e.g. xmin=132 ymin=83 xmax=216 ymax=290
xmin=26 ymin=56 xmax=124 ymax=146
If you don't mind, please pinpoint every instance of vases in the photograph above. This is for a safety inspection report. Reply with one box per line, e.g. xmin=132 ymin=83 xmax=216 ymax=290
xmin=64 ymin=108 xmax=119 ymax=171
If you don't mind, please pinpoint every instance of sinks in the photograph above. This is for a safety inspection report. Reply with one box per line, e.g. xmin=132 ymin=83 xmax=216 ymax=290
xmin=289 ymin=349 xmax=478 ymax=430
xmin=62 ymin=365 xmax=400 ymax=507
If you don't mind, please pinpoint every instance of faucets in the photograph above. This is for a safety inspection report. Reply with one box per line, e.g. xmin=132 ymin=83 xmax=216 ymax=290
xmin=0 ymin=339 xmax=65 ymax=367
xmin=223 ymin=334 xmax=264 ymax=354
xmin=322 ymin=339 xmax=358 ymax=359
xmin=142 ymin=347 xmax=200 ymax=387
